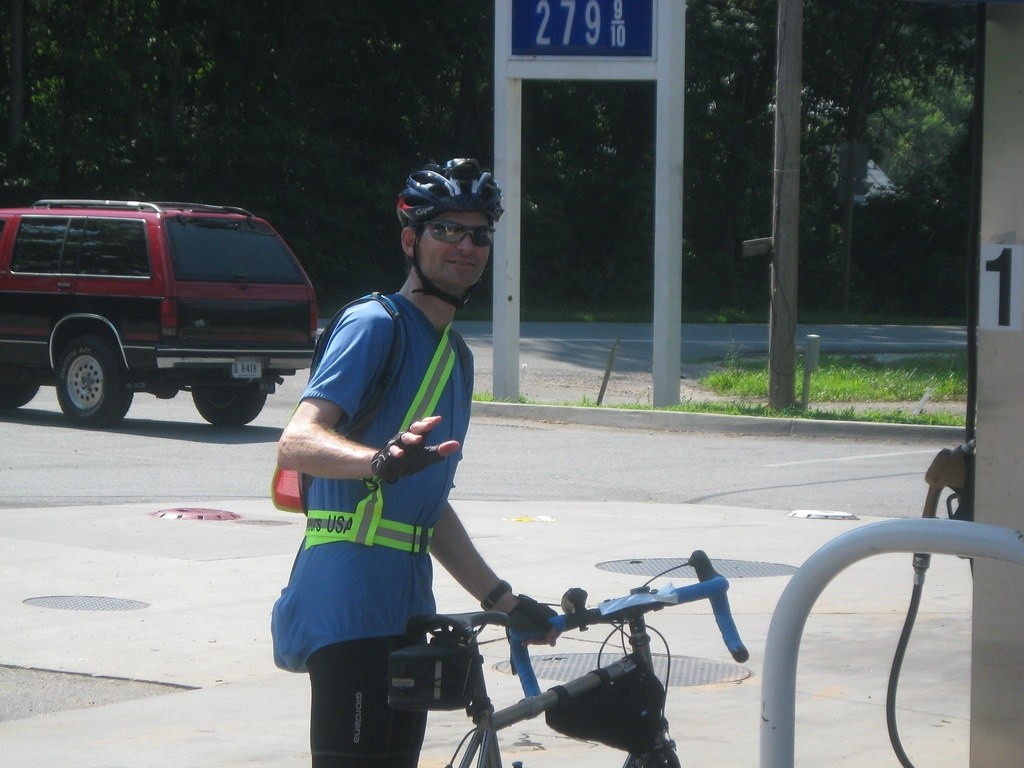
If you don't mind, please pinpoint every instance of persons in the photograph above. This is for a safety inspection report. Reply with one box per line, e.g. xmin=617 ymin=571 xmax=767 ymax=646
xmin=271 ymin=158 xmax=563 ymax=768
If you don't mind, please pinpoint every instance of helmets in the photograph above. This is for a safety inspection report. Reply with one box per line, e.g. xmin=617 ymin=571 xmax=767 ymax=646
xmin=396 ymin=156 xmax=504 ymax=225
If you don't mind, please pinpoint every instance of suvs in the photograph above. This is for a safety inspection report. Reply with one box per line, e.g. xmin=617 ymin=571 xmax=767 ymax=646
xmin=0 ymin=199 xmax=318 ymax=425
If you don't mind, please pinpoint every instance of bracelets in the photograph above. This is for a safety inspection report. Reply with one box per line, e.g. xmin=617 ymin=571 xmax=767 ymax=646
xmin=480 ymin=579 xmax=512 ymax=611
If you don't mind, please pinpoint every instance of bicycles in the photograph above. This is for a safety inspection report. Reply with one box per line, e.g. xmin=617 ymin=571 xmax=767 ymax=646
xmin=387 ymin=549 xmax=750 ymax=768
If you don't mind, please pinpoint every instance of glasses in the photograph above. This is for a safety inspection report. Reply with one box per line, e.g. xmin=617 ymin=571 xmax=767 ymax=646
xmin=408 ymin=219 xmax=496 ymax=246
